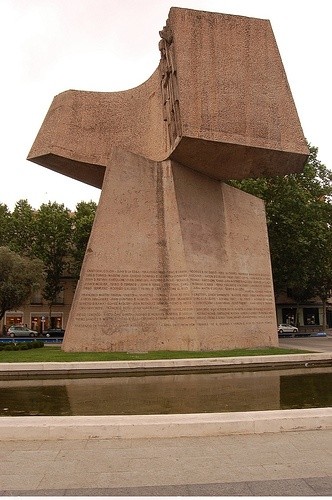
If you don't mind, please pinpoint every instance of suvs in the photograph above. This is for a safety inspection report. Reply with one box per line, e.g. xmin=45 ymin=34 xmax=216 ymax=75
xmin=7 ymin=325 xmax=38 ymax=338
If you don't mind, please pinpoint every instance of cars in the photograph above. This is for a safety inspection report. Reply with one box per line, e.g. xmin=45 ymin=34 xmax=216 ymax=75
xmin=277 ymin=324 xmax=298 ymax=334
xmin=42 ymin=328 xmax=65 ymax=338
